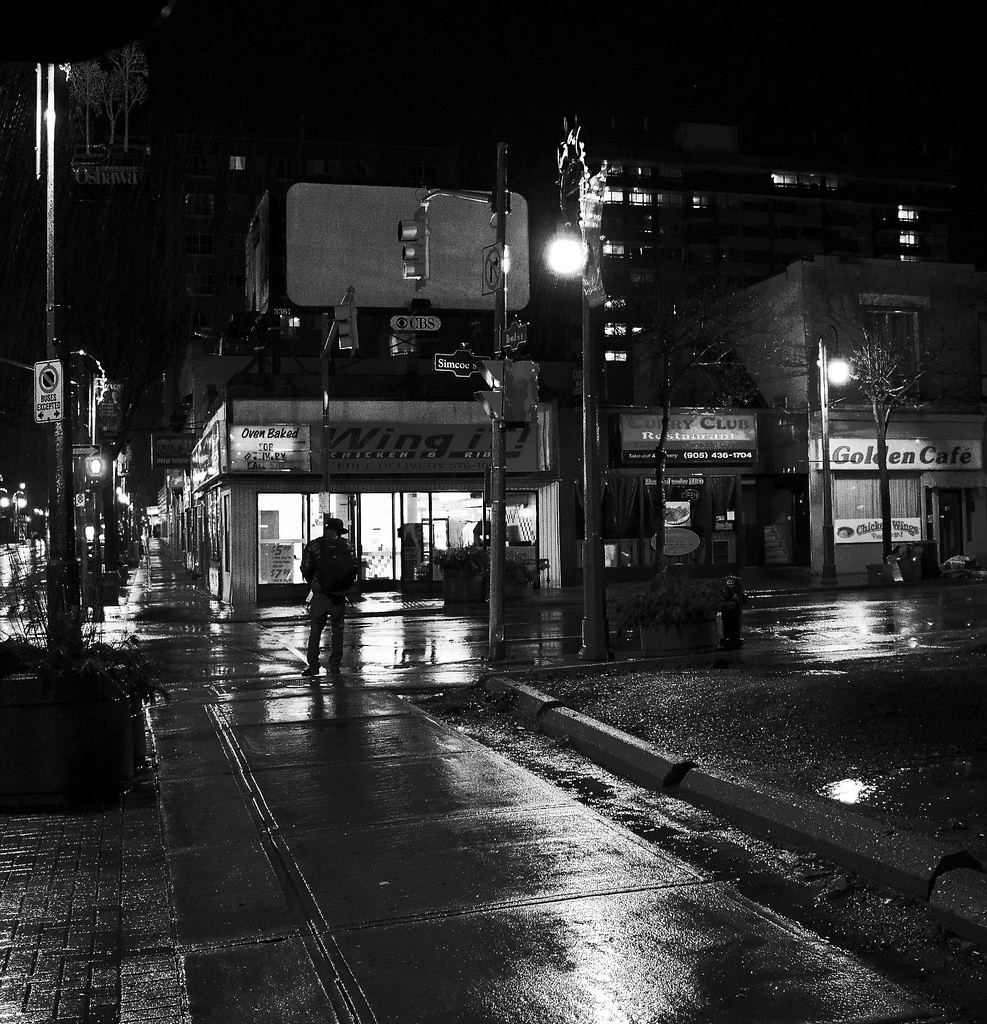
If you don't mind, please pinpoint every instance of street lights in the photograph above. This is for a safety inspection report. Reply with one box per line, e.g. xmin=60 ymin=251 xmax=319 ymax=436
xmin=818 ymin=339 xmax=853 ymax=587
xmin=542 ymin=224 xmax=609 ymax=661
xmin=85 ymin=456 xmax=104 ymax=622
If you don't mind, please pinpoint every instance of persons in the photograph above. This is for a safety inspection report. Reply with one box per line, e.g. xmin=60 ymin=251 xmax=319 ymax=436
xmin=300 ymin=518 xmax=356 ymax=678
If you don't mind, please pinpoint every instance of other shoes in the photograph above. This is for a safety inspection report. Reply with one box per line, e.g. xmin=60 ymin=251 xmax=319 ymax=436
xmin=331 ymin=668 xmax=341 ymax=674
xmin=302 ymin=668 xmax=319 ymax=676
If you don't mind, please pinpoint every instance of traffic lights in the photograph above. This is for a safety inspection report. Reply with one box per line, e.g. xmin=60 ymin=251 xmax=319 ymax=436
xmin=398 ymin=207 xmax=430 ymax=280
xmin=334 ymin=303 xmax=359 ymax=359
xmin=473 ymin=360 xmax=503 ymax=419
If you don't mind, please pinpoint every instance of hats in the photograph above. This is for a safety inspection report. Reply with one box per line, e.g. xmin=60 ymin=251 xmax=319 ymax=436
xmin=326 ymin=518 xmax=348 ymax=534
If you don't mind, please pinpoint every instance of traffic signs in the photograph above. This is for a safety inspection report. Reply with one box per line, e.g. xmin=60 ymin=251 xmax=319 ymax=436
xmin=72 ymin=443 xmax=101 ymax=458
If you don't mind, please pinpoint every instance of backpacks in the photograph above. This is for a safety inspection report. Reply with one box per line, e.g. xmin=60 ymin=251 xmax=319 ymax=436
xmin=317 ymin=538 xmax=355 ymax=592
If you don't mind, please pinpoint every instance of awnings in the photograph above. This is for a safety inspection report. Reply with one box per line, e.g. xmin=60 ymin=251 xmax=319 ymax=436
xmin=922 ymin=471 xmax=983 ymax=488
